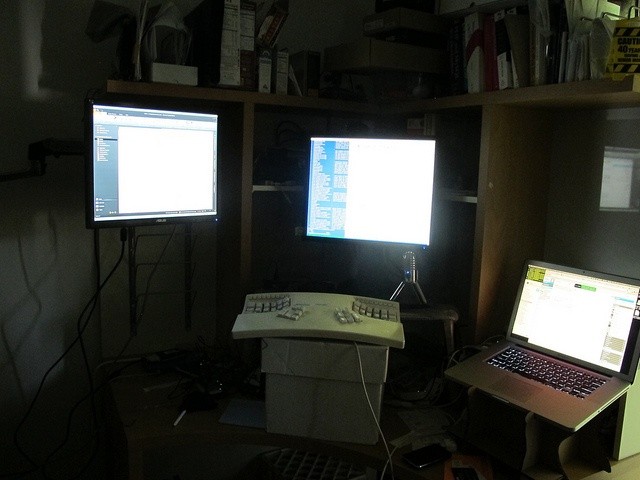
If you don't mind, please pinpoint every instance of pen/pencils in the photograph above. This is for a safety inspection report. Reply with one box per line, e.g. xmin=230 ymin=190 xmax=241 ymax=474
xmin=173 ymin=410 xmax=187 ymax=427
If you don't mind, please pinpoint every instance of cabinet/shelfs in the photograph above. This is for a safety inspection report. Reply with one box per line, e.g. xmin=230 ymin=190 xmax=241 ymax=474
xmin=93 ymin=77 xmax=640 ymax=353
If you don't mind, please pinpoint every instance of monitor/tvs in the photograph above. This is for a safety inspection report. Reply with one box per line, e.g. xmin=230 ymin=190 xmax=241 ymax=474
xmin=85 ymin=100 xmax=223 ymax=229
xmin=301 ymin=130 xmax=440 ymax=249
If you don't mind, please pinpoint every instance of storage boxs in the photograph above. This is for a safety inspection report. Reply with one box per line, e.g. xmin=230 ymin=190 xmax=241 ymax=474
xmin=258 ymin=334 xmax=390 ymax=447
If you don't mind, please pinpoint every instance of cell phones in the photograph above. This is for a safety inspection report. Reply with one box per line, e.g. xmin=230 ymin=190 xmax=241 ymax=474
xmin=401 ymin=444 xmax=451 ymax=470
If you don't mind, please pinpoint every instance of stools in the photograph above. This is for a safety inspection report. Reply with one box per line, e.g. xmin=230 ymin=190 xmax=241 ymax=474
xmin=400 ymin=302 xmax=461 ymax=362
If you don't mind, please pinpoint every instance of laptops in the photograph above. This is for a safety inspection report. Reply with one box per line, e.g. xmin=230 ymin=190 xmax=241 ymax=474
xmin=438 ymin=258 xmax=640 ymax=432
